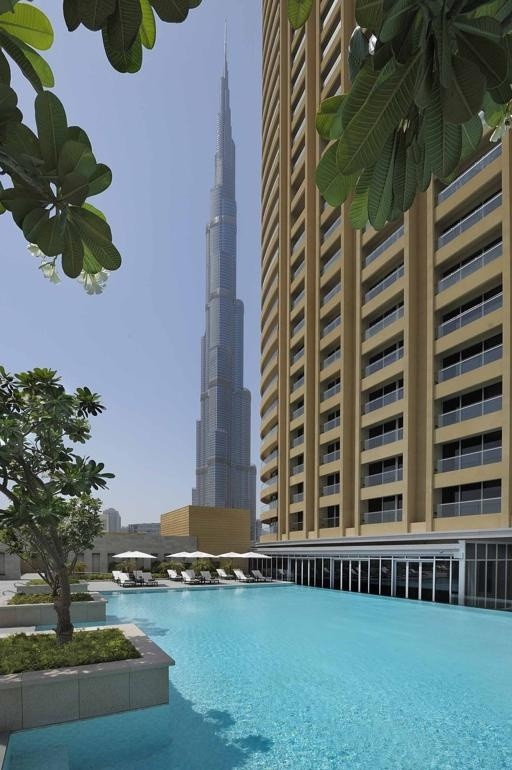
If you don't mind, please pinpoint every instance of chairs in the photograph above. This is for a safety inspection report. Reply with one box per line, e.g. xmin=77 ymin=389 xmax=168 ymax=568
xmin=167 ymin=570 xmax=183 ymax=583
xmin=252 ymin=570 xmax=272 ymax=582
xmin=112 ymin=571 xmax=122 ymax=583
xmin=216 ymin=569 xmax=234 ymax=580
xmin=181 ymin=572 xmax=200 ymax=584
xmin=233 ymin=569 xmax=250 ymax=579
xmin=141 ymin=572 xmax=158 ymax=586
xmin=118 ymin=573 xmax=135 ymax=587
xmin=234 ymin=571 xmax=256 ymax=582
xmin=277 ymin=568 xmax=295 ymax=582
xmin=133 ymin=571 xmax=142 ymax=584
xmin=186 ymin=570 xmax=203 ymax=582
xmin=200 ymin=571 xmax=219 ymax=584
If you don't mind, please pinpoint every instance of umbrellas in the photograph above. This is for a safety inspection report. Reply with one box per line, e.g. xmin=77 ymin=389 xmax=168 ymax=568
xmin=113 ymin=549 xmax=272 ymax=577
